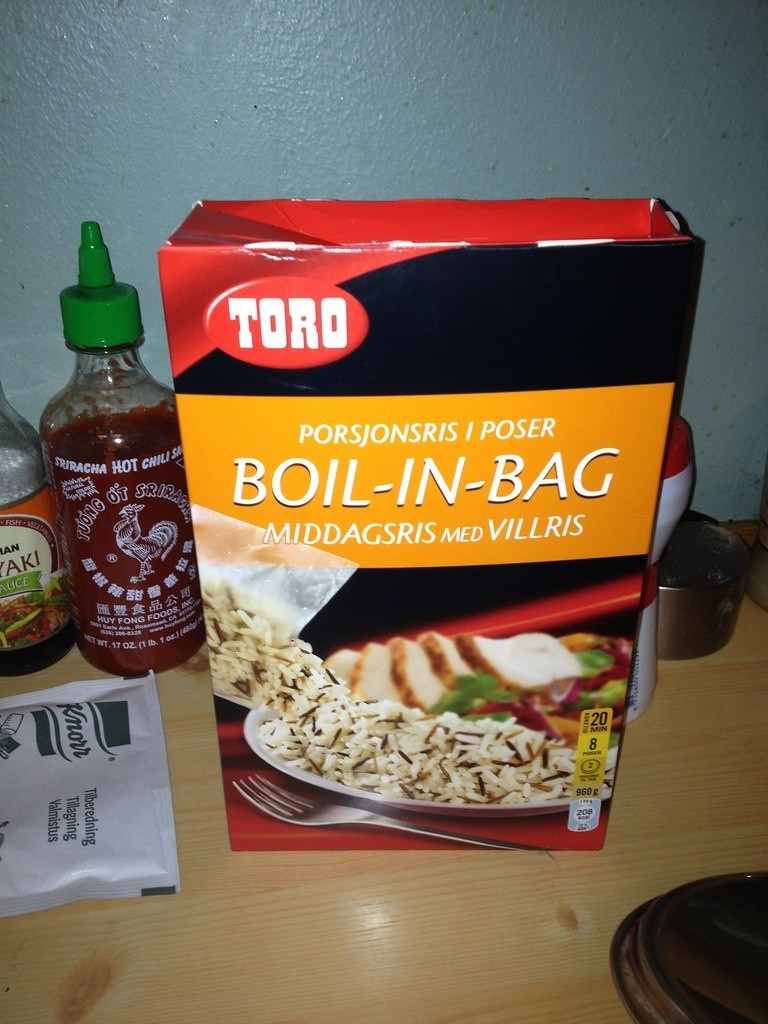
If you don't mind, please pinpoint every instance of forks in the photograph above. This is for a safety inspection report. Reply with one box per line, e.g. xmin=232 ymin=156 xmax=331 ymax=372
xmin=234 ymin=774 xmax=552 ymax=851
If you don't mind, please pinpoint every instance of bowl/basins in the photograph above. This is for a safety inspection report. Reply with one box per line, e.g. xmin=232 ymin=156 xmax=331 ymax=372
xmin=656 ymin=509 xmax=751 ymax=660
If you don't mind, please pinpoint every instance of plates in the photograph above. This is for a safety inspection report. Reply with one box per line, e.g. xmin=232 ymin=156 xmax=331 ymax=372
xmin=610 ymin=871 xmax=768 ymax=1024
xmin=243 ymin=705 xmax=612 ymax=817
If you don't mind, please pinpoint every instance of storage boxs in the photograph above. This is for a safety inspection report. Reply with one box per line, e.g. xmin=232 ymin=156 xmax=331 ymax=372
xmin=158 ymin=196 xmax=708 ymax=854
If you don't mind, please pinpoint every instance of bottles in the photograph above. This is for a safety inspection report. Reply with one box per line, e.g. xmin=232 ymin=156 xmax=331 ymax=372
xmin=37 ymin=220 xmax=204 ymax=678
xmin=0 ymin=379 xmax=76 ymax=675
xmin=624 ymin=413 xmax=694 ymax=726
